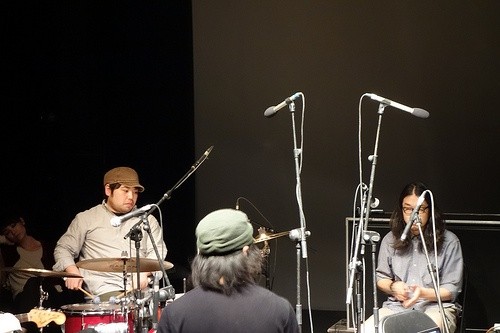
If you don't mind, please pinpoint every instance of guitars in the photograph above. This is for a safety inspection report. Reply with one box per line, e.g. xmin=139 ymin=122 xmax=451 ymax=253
xmin=14 ymin=305 xmax=67 ymax=329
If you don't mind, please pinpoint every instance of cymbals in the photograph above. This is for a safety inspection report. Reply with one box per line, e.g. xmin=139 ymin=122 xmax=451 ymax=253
xmin=254 ymin=229 xmax=291 ymax=243
xmin=76 ymin=256 xmax=174 ymax=273
xmin=0 ymin=267 xmax=84 ymax=279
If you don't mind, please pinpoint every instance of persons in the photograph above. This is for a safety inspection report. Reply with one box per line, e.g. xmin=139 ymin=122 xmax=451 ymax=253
xmin=0 ymin=211 xmax=55 ymax=312
xmin=53 ymin=166 xmax=169 ymax=304
xmin=375 ymin=182 xmax=464 ymax=333
xmin=156 ymin=208 xmax=299 ymax=333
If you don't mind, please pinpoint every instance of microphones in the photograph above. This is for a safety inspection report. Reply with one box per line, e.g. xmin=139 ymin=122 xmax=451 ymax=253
xmin=111 ymin=205 xmax=150 ymax=227
xmin=264 ymin=92 xmax=302 ymax=117
xmin=365 ymin=92 xmax=431 ymax=117
xmin=399 ymin=196 xmax=424 ymax=242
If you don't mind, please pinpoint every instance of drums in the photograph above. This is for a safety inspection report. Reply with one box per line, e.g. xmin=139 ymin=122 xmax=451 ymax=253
xmin=60 ymin=302 xmax=139 ymax=333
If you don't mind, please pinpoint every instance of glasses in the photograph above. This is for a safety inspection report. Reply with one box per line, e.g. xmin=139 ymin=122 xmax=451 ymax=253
xmin=402 ymin=206 xmax=428 ymax=214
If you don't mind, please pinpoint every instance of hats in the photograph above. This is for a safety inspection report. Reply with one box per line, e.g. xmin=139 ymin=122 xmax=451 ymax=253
xmin=103 ymin=167 xmax=144 ymax=193
xmin=195 ymin=208 xmax=254 ymax=256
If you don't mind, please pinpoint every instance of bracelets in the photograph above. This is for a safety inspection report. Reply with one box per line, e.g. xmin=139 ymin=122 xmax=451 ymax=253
xmin=389 ymin=280 xmax=396 ymax=294
